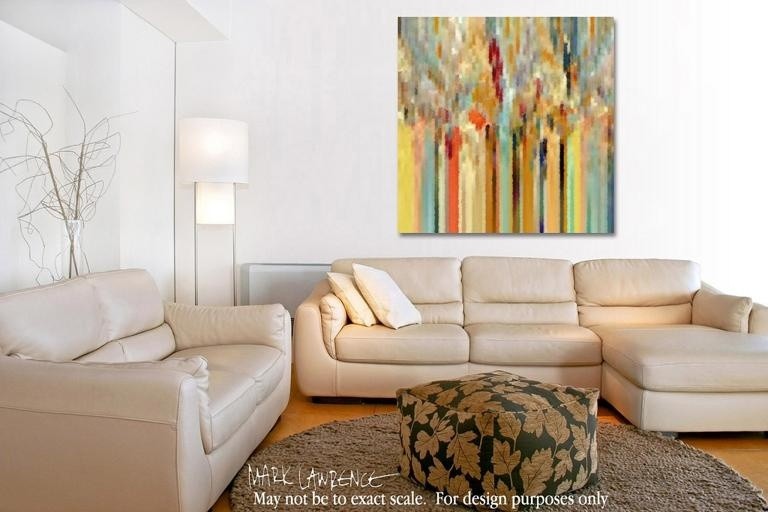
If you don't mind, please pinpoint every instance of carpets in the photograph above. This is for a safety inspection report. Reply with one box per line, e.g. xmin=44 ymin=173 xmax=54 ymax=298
xmin=233 ymin=410 xmax=766 ymax=511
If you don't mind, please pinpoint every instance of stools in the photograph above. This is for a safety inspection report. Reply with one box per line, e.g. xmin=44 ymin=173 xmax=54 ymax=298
xmin=397 ymin=368 xmax=600 ymax=511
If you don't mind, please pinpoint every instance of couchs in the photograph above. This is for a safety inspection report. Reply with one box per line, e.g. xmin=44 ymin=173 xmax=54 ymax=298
xmin=293 ymin=253 xmax=768 ymax=439
xmin=1 ymin=269 xmax=293 ymax=512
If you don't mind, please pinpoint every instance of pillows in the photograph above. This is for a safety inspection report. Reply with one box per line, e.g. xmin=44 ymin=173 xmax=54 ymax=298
xmin=326 ymin=261 xmax=421 ymax=329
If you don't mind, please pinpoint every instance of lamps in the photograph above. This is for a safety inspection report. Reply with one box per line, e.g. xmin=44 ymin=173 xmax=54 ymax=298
xmin=180 ymin=118 xmax=251 ymax=307
